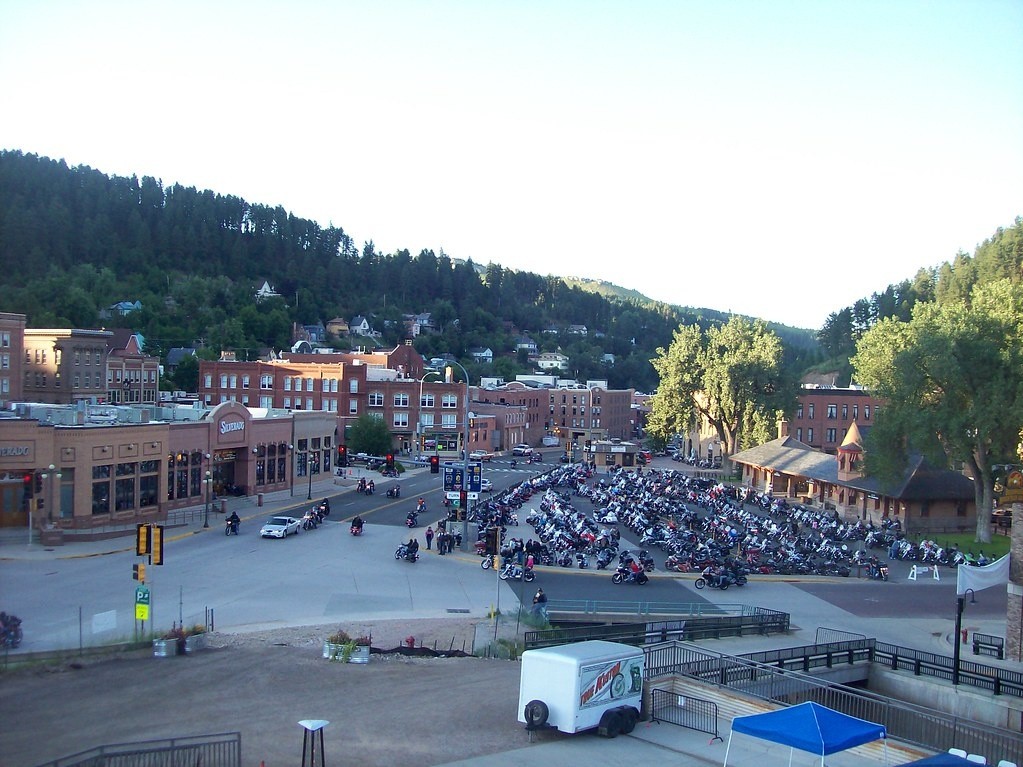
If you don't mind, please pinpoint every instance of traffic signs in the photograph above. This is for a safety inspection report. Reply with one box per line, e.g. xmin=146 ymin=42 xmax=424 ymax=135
xmin=135 ymin=587 xmax=152 ymax=606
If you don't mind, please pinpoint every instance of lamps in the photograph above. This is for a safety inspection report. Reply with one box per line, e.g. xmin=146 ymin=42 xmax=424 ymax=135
xmin=65 ymin=444 xmax=73 ymax=455
xmin=102 ymin=443 xmax=110 ymax=453
xmin=150 ymin=439 xmax=158 ymax=449
xmin=127 ymin=441 xmax=136 ymax=450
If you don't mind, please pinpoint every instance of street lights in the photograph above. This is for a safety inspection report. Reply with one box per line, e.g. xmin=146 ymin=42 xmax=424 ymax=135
xmin=203 ymin=471 xmax=211 ymax=528
xmin=307 ymin=454 xmax=315 ymax=498
xmin=573 ymin=383 xmax=596 ymax=468
xmin=431 ymin=357 xmax=468 ymax=521
xmin=951 ymin=586 xmax=981 ymax=684
xmin=417 ymin=370 xmax=440 ymax=463
xmin=287 ymin=412 xmax=295 ymax=497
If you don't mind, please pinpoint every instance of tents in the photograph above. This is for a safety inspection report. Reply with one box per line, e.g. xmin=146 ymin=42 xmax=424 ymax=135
xmin=723 ymin=701 xmax=890 ymax=767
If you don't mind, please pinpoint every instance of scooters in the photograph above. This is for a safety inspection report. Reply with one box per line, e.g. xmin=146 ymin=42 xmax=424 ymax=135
xmin=405 ymin=518 xmax=418 ymax=529
xmin=355 ymin=484 xmax=401 ymax=499
xmin=417 ymin=503 xmax=427 ymax=512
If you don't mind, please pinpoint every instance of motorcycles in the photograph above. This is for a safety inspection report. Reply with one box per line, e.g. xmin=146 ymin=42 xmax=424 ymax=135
xmin=393 ymin=542 xmax=420 ymax=563
xmin=302 ymin=502 xmax=330 ymax=530
xmin=224 ymin=515 xmax=241 ymax=536
xmin=478 ymin=433 xmax=998 ymax=591
xmin=350 ymin=518 xmax=367 ymax=536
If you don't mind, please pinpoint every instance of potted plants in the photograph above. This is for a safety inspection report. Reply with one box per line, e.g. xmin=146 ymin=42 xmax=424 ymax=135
xmin=150 ymin=624 xmax=209 ymax=659
xmin=322 ymin=628 xmax=371 ymax=665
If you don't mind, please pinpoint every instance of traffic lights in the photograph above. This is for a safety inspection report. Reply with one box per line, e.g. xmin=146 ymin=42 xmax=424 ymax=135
xmin=339 ymin=445 xmax=347 ymax=468
xmin=23 ymin=474 xmax=34 ymax=500
xmin=587 ymin=440 xmax=591 ymax=448
xmin=431 ymin=456 xmax=439 ymax=473
xmin=460 ymin=491 xmax=467 ymax=503
xmin=131 ymin=563 xmax=144 ymax=581
xmin=33 ymin=497 xmax=44 ymax=510
xmin=545 ymin=422 xmax=549 ymax=430
xmin=585 ymin=440 xmax=588 ymax=447
xmin=386 ymin=451 xmax=395 ymax=469
xmin=35 ymin=473 xmax=43 ymax=493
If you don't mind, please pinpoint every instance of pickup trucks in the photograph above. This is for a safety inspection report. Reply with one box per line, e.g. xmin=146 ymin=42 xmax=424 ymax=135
xmin=513 ymin=444 xmax=534 ymax=456
xmin=470 ymin=449 xmax=494 ymax=463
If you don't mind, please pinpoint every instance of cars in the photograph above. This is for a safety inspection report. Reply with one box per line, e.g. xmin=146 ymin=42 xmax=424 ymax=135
xmin=481 ymin=479 xmax=493 ymax=492
xmin=261 ymin=517 xmax=301 ymax=539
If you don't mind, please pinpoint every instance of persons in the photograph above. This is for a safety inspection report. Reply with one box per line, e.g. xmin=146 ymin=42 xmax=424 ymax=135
xmin=601 ymin=525 xmax=610 ymax=541
xmin=791 ymin=520 xmax=800 ymax=534
xmin=425 ymin=526 xmax=434 ymax=550
xmin=509 ymin=551 xmax=533 ymax=577
xmin=401 ymin=538 xmax=419 ymax=560
xmin=352 ymin=516 xmax=362 ymax=528
xmin=532 ymin=587 xmax=547 ymax=616
xmin=768 ymin=482 xmax=773 ymax=497
xmin=852 ymin=516 xmax=958 ymax=580
xmin=407 ymin=498 xmax=428 ymax=525
xmin=0 ymin=611 xmax=14 ymax=640
xmin=689 ymin=534 xmax=697 ymax=544
xmin=434 ymin=524 xmax=456 ymax=555
xmin=225 ymin=511 xmax=240 ymax=532
xmin=979 ymin=549 xmax=986 ymax=562
xmin=834 ymin=510 xmax=839 ymax=517
xmin=309 ymin=497 xmax=329 ymax=529
xmin=526 ymin=539 xmax=541 ymax=554
xmin=623 ymin=559 xmax=645 ymax=585
xmin=415 ymin=455 xmax=429 ymax=467
xmin=606 ymin=464 xmax=616 ymax=478
xmin=510 ymin=537 xmax=524 ymax=554
xmin=564 ymin=490 xmax=570 ymax=502
xmin=717 ymin=564 xmax=729 ymax=586
xmin=360 ymin=476 xmax=375 ymax=491
xmin=610 ymin=526 xmax=618 ymax=541
